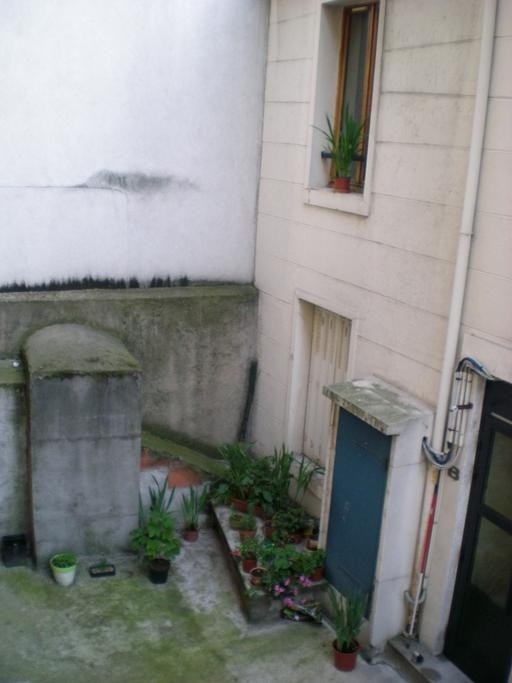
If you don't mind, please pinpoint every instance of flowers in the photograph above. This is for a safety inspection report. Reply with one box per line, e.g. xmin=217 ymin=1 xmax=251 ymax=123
xmin=274 ymin=574 xmax=319 ymax=622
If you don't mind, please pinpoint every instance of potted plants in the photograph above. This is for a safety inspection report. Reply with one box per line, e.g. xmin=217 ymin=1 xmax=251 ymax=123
xmin=51 ymin=552 xmax=78 ymax=587
xmin=312 ymin=105 xmax=365 ymax=193
xmin=84 ymin=525 xmax=118 ymax=577
xmin=216 ymin=442 xmax=329 ymax=590
xmin=316 ymin=586 xmax=361 ymax=673
xmin=129 ymin=502 xmax=184 ymax=584
xmin=182 ymin=484 xmax=208 ymax=543
